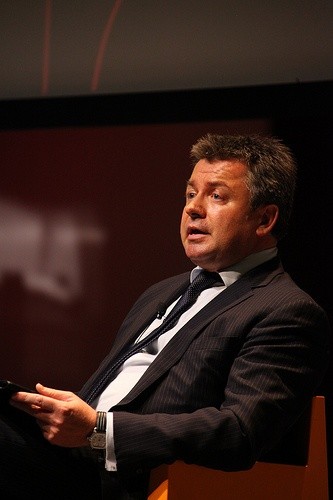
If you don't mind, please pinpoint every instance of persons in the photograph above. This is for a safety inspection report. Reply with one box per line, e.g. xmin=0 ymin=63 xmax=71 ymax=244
xmin=0 ymin=131 xmax=333 ymax=499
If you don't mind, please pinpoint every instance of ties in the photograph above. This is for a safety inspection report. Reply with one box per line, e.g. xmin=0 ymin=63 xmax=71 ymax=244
xmin=85 ymin=270 xmax=223 ymax=402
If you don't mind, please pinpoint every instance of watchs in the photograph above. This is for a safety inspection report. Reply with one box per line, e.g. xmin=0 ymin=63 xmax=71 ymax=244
xmin=89 ymin=411 xmax=106 ymax=461
